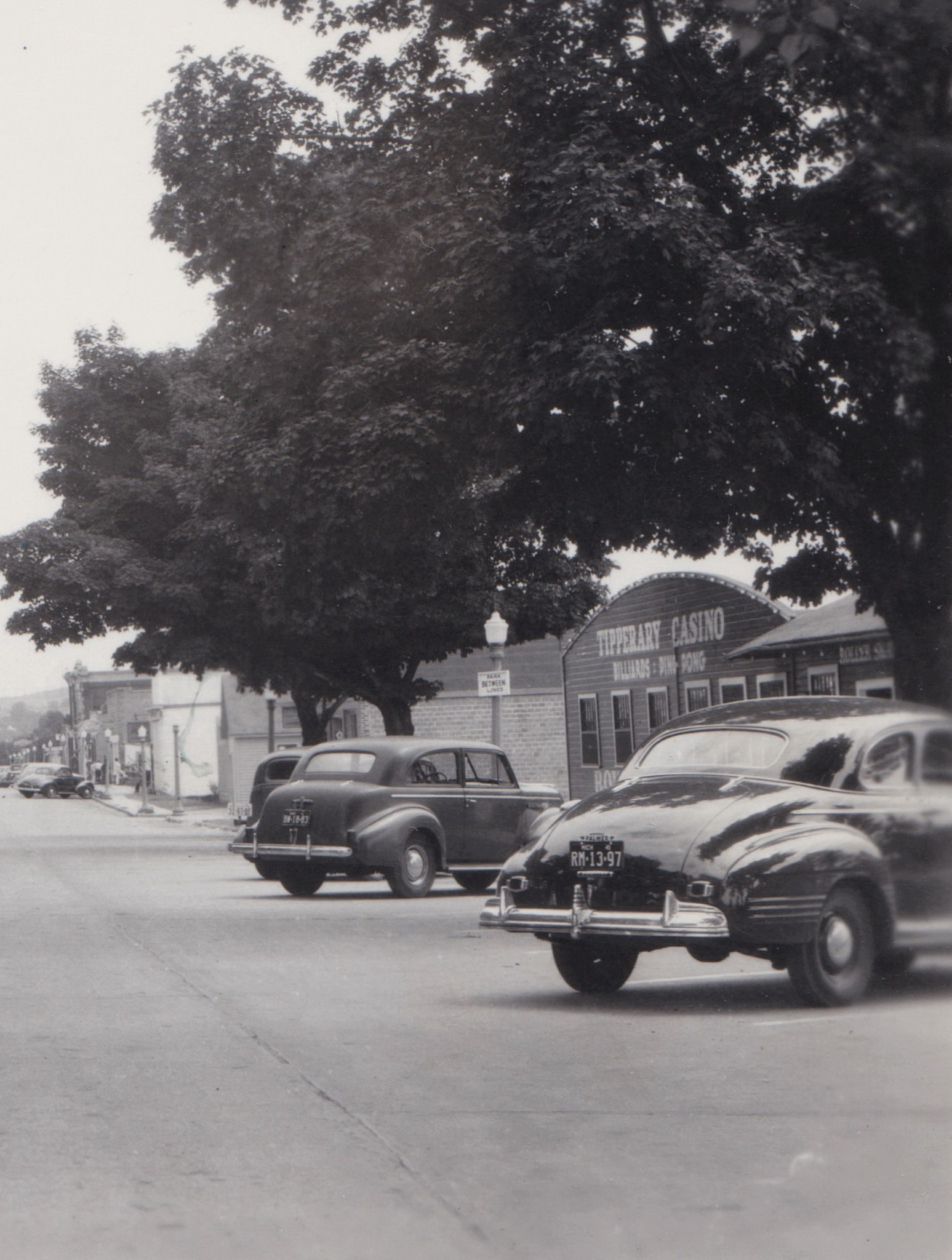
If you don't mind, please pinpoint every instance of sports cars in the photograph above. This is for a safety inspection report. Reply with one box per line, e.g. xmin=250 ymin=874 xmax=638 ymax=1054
xmin=228 ymin=736 xmax=564 ymax=900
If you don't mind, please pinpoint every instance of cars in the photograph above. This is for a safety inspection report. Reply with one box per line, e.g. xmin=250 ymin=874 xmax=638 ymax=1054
xmin=0 ymin=762 xmax=95 ymax=800
xmin=233 ymin=745 xmax=315 ymax=827
xmin=477 ymin=693 xmax=952 ymax=1008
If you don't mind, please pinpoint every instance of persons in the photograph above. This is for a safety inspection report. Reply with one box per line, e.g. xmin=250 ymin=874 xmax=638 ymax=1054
xmin=113 ymin=757 xmax=127 ymax=785
xmin=90 ymin=760 xmax=104 ymax=783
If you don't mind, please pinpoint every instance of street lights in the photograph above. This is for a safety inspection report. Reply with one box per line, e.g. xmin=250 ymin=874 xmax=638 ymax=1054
xmin=170 ymin=709 xmax=187 ymax=816
xmin=9 ymin=748 xmax=30 ymax=765
xmin=61 ymin=733 xmax=66 ymax=764
xmin=102 ymin=727 xmax=112 ymax=800
xmin=32 ymin=746 xmax=37 ymax=762
xmin=80 ymin=729 xmax=88 ymax=775
xmin=482 ymin=609 xmax=509 ymax=781
xmin=48 ymin=740 xmax=53 ymax=762
xmin=137 ymin=724 xmax=154 ymax=812
xmin=42 ymin=743 xmax=46 ymax=762
